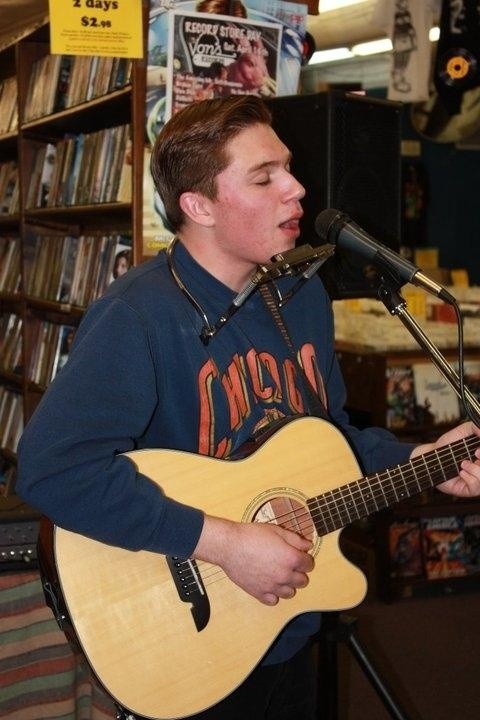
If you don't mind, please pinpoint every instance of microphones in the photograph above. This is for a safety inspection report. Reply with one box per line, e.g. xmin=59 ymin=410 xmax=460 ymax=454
xmin=315 ymin=208 xmax=457 ymax=302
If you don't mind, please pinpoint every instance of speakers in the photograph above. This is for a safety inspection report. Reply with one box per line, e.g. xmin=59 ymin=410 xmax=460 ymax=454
xmin=264 ymin=90 xmax=403 ymax=299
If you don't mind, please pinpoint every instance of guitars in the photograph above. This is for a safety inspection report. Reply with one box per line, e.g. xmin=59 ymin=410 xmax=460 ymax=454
xmin=35 ymin=415 xmax=479 ymax=718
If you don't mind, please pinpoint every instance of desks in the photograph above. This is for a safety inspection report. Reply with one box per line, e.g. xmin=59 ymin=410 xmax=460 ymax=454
xmin=325 ymin=288 xmax=480 ymax=606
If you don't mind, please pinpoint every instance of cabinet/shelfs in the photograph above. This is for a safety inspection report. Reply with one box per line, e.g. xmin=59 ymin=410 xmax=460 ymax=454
xmin=0 ymin=1 xmax=321 ymax=548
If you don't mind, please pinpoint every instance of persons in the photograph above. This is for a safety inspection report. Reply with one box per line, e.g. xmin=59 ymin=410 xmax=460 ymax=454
xmin=197 ymin=62 xmax=229 ymax=104
xmin=14 ymin=93 xmax=479 ymax=720
xmin=196 ymin=0 xmax=247 ymax=19
